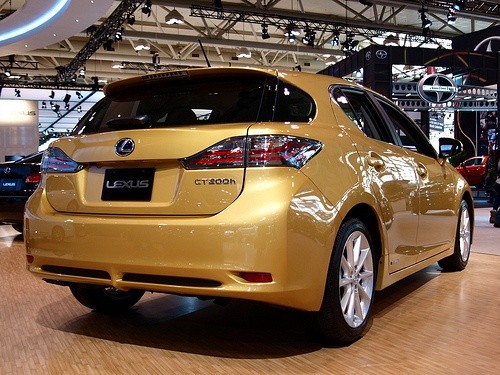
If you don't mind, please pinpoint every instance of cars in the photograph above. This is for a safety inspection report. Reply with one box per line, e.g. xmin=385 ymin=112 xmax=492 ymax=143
xmin=455 ymin=155 xmax=489 ymax=190
xmin=22 ymin=66 xmax=477 ymax=334
xmin=0 ymin=150 xmax=43 ymax=232
